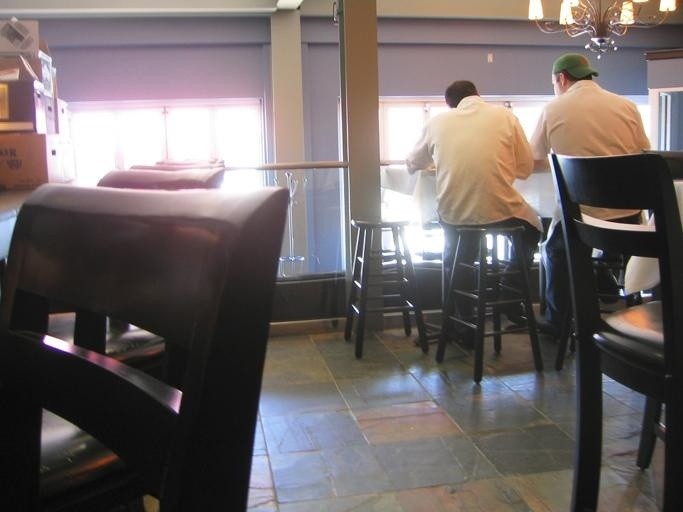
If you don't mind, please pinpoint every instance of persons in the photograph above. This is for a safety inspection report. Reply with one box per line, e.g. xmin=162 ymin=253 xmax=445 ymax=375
xmin=404 ymin=79 xmax=545 ymax=331
xmin=526 ymin=52 xmax=652 ymax=341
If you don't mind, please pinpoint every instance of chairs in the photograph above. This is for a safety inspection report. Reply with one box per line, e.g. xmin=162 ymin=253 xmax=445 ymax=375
xmin=543 ymin=143 xmax=680 ymax=511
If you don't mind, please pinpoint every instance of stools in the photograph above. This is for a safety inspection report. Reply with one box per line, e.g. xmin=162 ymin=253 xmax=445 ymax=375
xmin=343 ymin=217 xmax=429 ymax=362
xmin=434 ymin=225 xmax=543 ymax=384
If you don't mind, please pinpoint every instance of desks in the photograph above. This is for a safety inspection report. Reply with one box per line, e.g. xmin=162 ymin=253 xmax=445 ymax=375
xmin=259 ymin=159 xmax=429 ymax=177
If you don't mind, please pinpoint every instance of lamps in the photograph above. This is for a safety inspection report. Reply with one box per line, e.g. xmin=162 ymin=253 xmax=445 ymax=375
xmin=522 ymin=0 xmax=681 ymax=62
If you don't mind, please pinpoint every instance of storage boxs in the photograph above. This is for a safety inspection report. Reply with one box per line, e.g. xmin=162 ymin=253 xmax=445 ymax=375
xmin=0 ymin=17 xmax=72 ymax=189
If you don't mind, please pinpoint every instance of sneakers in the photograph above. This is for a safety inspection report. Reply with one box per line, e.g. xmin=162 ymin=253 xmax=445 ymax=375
xmin=538 ymin=321 xmax=561 ymax=337
xmin=497 ymin=298 xmax=527 ymax=322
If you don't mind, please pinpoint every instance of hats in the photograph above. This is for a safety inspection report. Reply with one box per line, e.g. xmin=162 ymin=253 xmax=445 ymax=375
xmin=553 ymin=55 xmax=599 ymax=79
